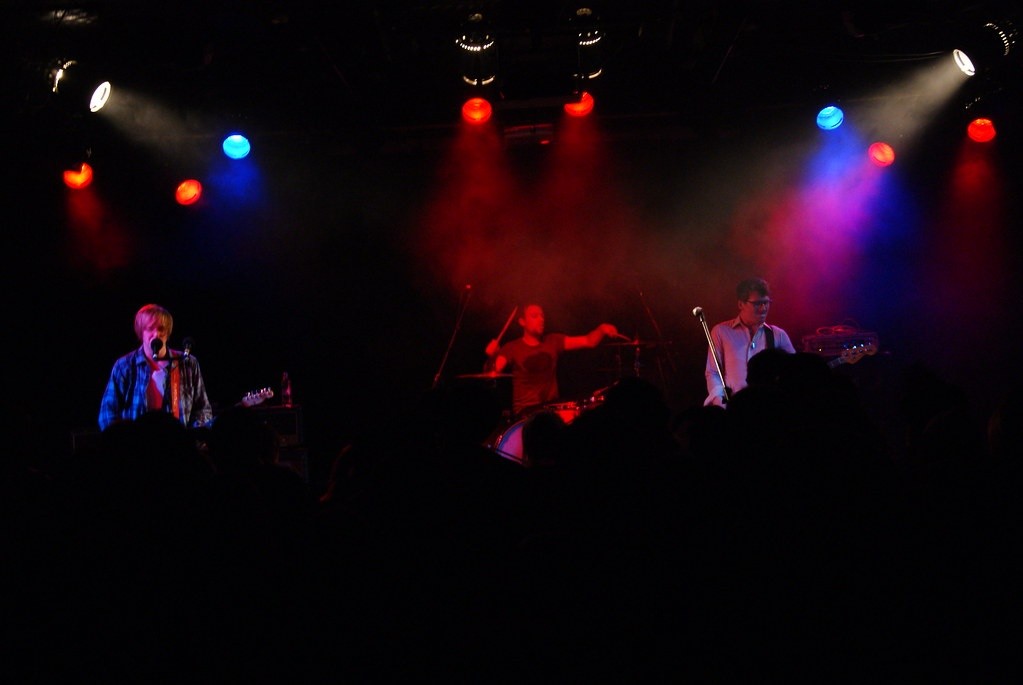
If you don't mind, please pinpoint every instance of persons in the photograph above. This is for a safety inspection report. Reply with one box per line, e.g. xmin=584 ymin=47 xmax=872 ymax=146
xmin=701 ymin=276 xmax=796 ymax=407
xmin=0 ymin=349 xmax=1023 ymax=685
xmin=95 ymin=303 xmax=215 ymax=431
xmin=484 ymin=303 xmax=618 ymax=411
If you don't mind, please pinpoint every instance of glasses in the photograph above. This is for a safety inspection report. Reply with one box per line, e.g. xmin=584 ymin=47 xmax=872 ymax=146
xmin=742 ymin=299 xmax=772 ymax=306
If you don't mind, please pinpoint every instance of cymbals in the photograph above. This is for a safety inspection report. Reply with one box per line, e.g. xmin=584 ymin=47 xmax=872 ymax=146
xmin=607 ymin=341 xmax=657 ymax=347
xmin=452 ymin=374 xmax=515 ymax=381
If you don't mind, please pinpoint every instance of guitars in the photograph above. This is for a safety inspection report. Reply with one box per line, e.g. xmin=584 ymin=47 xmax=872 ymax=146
xmin=828 ymin=338 xmax=878 ymax=368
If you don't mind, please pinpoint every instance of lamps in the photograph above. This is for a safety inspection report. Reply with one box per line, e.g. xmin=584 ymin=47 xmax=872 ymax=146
xmin=41 ymin=58 xmax=112 ymax=112
xmin=954 ymin=21 xmax=1011 ymax=77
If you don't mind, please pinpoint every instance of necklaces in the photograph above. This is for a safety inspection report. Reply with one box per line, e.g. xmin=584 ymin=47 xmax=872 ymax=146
xmin=735 ymin=325 xmax=764 ymax=348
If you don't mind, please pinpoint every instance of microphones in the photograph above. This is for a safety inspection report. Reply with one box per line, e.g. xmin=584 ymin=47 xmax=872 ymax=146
xmin=150 ymin=338 xmax=164 ymax=360
xmin=182 ymin=336 xmax=195 ymax=358
xmin=692 ymin=306 xmax=705 ymax=323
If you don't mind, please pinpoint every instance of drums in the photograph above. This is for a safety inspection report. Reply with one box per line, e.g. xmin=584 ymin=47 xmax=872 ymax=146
xmin=480 ymin=394 xmax=605 ymax=468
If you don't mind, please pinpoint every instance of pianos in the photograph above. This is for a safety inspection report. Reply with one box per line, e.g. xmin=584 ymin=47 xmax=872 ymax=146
xmin=68 ymin=431 xmax=217 ymax=465
